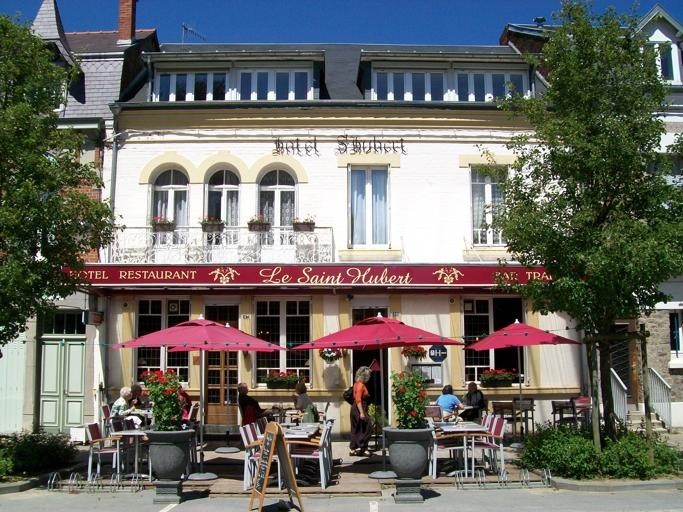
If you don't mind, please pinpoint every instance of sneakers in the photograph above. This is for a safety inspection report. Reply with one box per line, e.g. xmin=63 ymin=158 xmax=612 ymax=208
xmin=350 ymin=451 xmax=359 ymax=456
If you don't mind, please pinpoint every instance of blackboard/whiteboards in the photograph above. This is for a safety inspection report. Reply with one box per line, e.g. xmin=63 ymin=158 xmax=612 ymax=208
xmin=253 ymin=421 xmax=278 ymax=498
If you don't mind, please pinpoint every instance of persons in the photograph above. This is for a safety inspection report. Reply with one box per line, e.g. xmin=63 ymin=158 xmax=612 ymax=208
xmin=174 ymin=383 xmax=192 ymax=419
xmin=237 ymin=383 xmax=270 ymax=420
xmin=291 ymin=383 xmax=314 ymax=413
xmin=349 ymin=366 xmax=374 ymax=456
xmin=434 ymin=384 xmax=463 ymax=420
xmin=461 ymin=381 xmax=484 ymax=420
xmin=110 ymin=386 xmax=148 ymax=443
xmin=129 ymin=383 xmax=145 ymax=409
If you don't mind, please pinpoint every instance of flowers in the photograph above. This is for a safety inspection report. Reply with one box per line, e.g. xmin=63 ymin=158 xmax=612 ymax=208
xmin=389 ymin=366 xmax=429 ymax=428
xmin=266 ymin=368 xmax=302 ymax=383
xmin=400 ymin=346 xmax=427 ymax=360
xmin=140 ymin=368 xmax=189 ymax=431
xmin=480 ymin=367 xmax=513 ymax=378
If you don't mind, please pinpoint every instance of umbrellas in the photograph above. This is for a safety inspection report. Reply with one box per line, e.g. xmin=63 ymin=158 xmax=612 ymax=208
xmin=291 ymin=312 xmax=470 ymax=472
xmin=461 ymin=319 xmax=582 ymax=443
xmin=111 ymin=312 xmax=290 ymax=474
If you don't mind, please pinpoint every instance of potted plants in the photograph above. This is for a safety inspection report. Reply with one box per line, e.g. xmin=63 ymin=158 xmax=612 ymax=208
xmin=247 ymin=214 xmax=270 ymax=232
xmin=200 ymin=216 xmax=224 ymax=232
xmin=291 ymin=212 xmax=315 ymax=232
xmin=151 ymin=214 xmax=176 ymax=232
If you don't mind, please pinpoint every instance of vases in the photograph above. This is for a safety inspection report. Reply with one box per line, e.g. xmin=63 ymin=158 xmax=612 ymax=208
xmin=266 ymin=382 xmax=297 ymax=390
xmin=480 ymin=377 xmax=512 ymax=388
xmin=381 ymin=426 xmax=433 ymax=480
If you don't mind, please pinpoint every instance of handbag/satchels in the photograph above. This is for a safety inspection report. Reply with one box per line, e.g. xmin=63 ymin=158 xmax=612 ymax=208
xmin=344 ymin=386 xmax=353 ymax=404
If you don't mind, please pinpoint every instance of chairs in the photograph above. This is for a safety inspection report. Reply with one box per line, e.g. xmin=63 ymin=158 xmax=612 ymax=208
xmin=424 ymin=396 xmax=507 ymax=481
xmin=238 ymin=400 xmax=337 ymax=490
xmin=492 ymin=397 xmax=591 ymax=436
xmin=81 ymin=403 xmax=200 ymax=480
